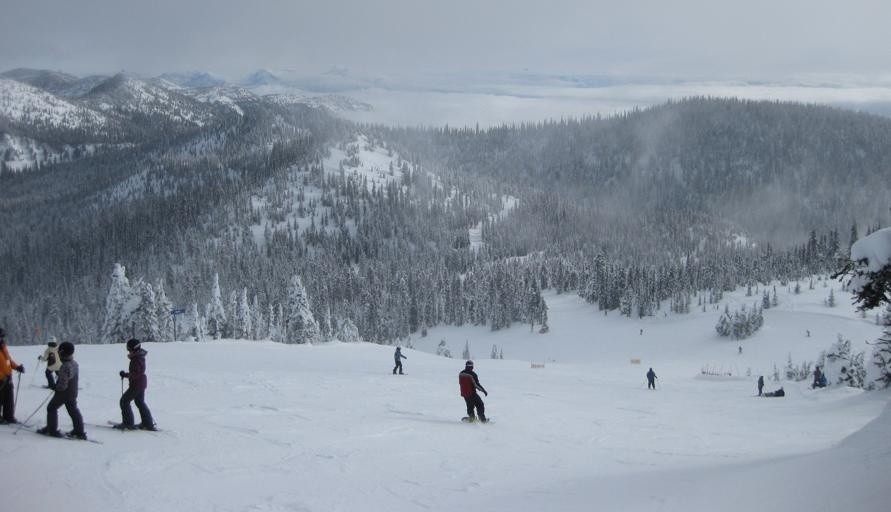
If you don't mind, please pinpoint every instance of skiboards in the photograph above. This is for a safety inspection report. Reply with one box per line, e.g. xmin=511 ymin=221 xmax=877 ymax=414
xmin=0 ymin=420 xmax=159 ymax=444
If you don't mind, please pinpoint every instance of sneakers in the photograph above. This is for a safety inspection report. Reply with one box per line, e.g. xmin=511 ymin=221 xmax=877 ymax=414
xmin=71 ymin=430 xmax=86 ymax=437
xmin=137 ymin=422 xmax=154 ymax=430
xmin=115 ymin=422 xmax=136 ymax=429
xmin=42 ymin=427 xmax=58 ymax=435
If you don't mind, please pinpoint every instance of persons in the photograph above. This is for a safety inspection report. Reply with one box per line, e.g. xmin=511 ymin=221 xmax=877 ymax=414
xmin=111 ymin=338 xmax=154 ymax=431
xmin=646 ymin=367 xmax=658 ymax=390
xmin=392 ymin=345 xmax=407 ymax=376
xmin=815 ymin=371 xmax=827 ymax=388
xmin=762 ymin=386 xmax=785 ymax=398
xmin=35 ymin=341 xmax=88 ymax=440
xmin=457 ymin=360 xmax=489 ymax=424
xmin=756 ymin=375 xmax=764 ymax=396
xmin=37 ymin=341 xmax=61 ymax=390
xmin=0 ymin=326 xmax=26 ymax=424
xmin=812 ymin=365 xmax=823 ymax=392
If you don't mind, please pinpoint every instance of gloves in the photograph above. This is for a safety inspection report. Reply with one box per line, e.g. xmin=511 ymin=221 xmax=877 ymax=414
xmin=49 ymin=383 xmax=56 ymax=390
xmin=17 ymin=365 xmax=24 ymax=372
xmin=120 ymin=370 xmax=128 ymax=378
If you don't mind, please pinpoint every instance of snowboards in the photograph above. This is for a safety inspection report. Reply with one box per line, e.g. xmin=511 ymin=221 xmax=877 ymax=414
xmin=462 ymin=416 xmax=487 ymax=422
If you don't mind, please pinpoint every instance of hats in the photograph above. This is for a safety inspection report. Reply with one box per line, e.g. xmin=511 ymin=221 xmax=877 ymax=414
xmin=127 ymin=339 xmax=141 ymax=351
xmin=47 ymin=336 xmax=56 ymax=343
xmin=58 ymin=342 xmax=75 ymax=359
